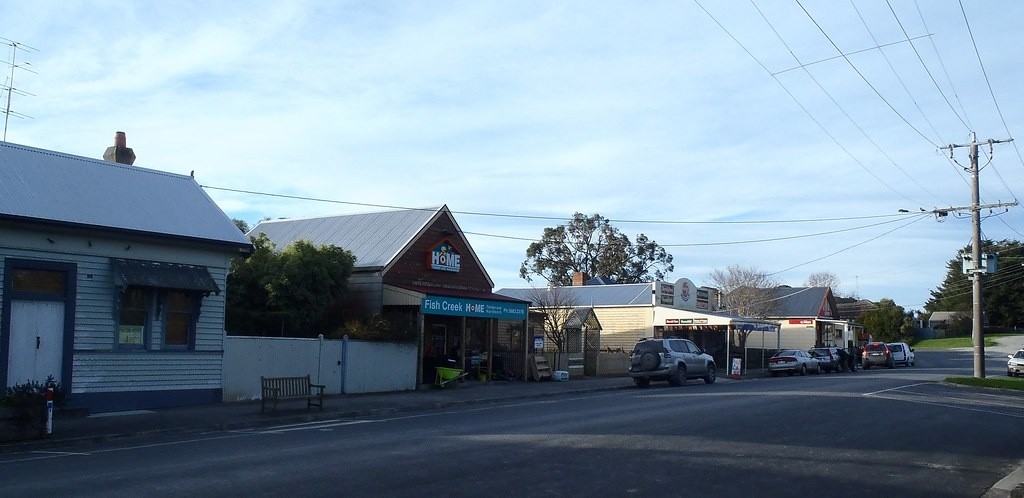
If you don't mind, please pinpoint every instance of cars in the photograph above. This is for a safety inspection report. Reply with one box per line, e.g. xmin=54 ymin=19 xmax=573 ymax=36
xmin=1006 ymin=348 xmax=1024 ymax=377
xmin=767 ymin=348 xmax=821 ymax=377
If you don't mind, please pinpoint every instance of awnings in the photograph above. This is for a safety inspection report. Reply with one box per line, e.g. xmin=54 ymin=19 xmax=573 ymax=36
xmin=108 ymin=257 xmax=221 ymax=297
xmin=651 ymin=306 xmax=781 ymax=333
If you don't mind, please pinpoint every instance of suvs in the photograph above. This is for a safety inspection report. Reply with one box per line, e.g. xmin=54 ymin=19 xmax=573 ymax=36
xmin=808 ymin=346 xmax=854 ymax=373
xmin=860 ymin=341 xmax=896 ymax=370
xmin=887 ymin=342 xmax=916 ymax=367
xmin=628 ymin=336 xmax=717 ymax=388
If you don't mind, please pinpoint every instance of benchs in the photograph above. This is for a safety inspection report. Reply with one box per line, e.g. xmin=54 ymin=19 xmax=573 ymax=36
xmin=261 ymin=374 xmax=326 ymax=413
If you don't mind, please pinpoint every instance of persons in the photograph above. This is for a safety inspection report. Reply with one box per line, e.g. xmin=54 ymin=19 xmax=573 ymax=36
xmin=835 ymin=347 xmax=851 ymax=373
